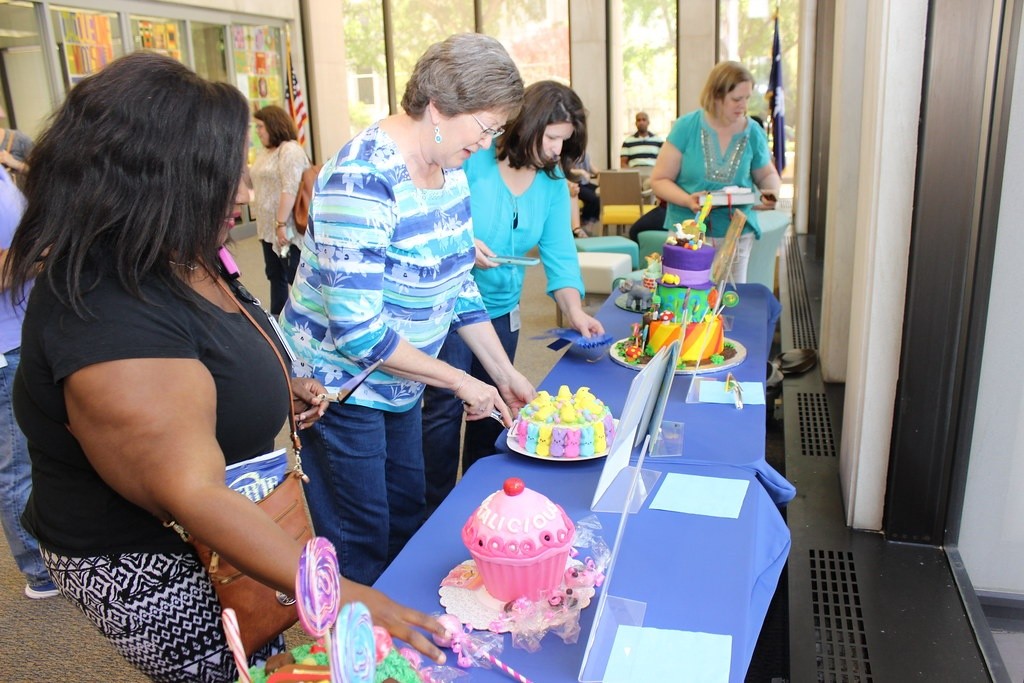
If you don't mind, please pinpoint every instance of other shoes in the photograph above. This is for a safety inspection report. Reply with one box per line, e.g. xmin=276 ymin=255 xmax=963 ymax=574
xmin=24 ymin=580 xmax=61 ymax=600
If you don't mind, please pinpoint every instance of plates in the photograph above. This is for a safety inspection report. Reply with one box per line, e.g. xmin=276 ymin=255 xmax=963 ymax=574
xmin=506 ymin=419 xmax=619 ymax=462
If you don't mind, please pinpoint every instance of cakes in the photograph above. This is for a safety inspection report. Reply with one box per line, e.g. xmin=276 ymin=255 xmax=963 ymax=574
xmin=514 ymin=384 xmax=618 ymax=458
xmin=617 ymin=289 xmax=739 ymax=368
xmin=660 ymin=219 xmax=714 ymax=285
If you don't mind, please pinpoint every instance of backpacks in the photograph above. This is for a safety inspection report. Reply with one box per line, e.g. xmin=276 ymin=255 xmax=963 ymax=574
xmin=276 ymin=141 xmax=323 ymax=236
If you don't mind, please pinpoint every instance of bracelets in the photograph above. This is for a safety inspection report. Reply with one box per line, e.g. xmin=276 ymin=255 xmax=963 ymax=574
xmin=454 ymin=370 xmax=467 ymax=398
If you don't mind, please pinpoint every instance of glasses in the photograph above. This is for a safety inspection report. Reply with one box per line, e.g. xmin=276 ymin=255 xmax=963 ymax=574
xmin=468 ymin=112 xmax=506 ymax=140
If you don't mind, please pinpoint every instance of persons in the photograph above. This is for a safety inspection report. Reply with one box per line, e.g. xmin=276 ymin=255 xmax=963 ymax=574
xmin=277 ymin=30 xmax=541 ymax=588
xmin=619 ymin=112 xmax=666 ymax=205
xmin=0 ymin=127 xmax=60 ymax=598
xmin=566 ymin=153 xmax=601 ymax=238
xmin=648 ymin=60 xmax=781 ymax=284
xmin=423 ymin=81 xmax=605 ymax=519
xmin=0 ymin=51 xmax=452 ymax=683
xmin=250 ymin=105 xmax=311 ymax=323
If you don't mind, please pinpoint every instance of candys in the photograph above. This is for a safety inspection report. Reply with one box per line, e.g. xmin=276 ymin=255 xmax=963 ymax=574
xmin=220 ymin=536 xmax=429 ymax=683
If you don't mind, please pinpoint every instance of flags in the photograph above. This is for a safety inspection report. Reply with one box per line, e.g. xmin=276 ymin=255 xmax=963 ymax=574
xmin=284 ymin=38 xmax=306 ymax=147
xmin=764 ymin=7 xmax=786 ymax=179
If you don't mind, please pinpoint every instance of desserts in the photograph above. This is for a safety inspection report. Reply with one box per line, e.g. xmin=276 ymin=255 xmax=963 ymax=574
xmin=461 ymin=479 xmax=575 ymax=606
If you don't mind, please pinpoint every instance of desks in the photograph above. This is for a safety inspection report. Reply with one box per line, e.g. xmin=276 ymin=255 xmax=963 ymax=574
xmin=747 ymin=210 xmax=790 ymax=294
xmin=373 ymin=283 xmax=795 ymax=683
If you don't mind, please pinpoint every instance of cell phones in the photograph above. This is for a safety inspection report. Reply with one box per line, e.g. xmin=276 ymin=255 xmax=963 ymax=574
xmin=488 ymin=255 xmax=540 ymax=265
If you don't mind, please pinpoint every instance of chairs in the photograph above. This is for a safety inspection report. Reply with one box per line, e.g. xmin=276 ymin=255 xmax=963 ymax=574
xmin=578 ymin=170 xmax=661 ymax=236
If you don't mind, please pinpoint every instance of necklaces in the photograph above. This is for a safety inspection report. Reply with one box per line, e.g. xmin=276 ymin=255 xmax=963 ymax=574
xmin=169 ymin=259 xmax=199 ymax=270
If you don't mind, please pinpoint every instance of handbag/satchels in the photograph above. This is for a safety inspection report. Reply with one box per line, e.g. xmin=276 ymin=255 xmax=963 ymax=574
xmin=159 ymin=435 xmax=336 ymax=660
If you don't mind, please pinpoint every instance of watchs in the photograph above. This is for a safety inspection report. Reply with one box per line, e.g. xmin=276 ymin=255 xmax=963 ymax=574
xmin=275 ymin=221 xmax=287 ymax=228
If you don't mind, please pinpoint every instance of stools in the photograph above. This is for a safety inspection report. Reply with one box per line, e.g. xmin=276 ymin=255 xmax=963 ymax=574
xmin=556 ymin=230 xmax=668 ymax=326
